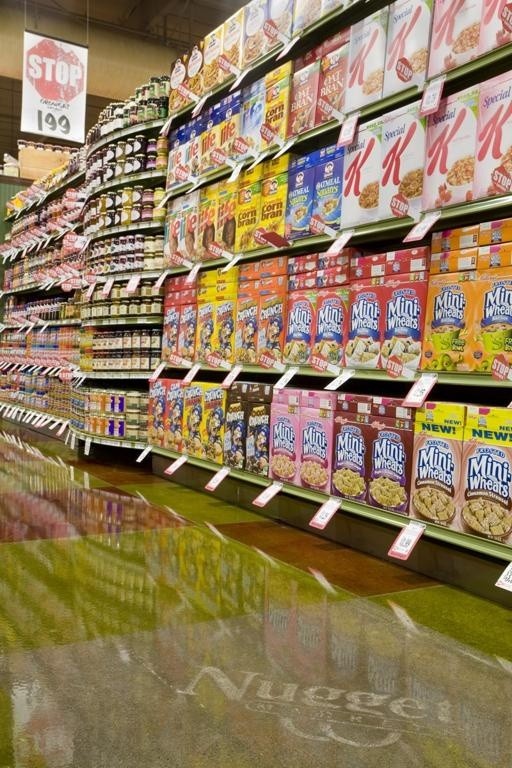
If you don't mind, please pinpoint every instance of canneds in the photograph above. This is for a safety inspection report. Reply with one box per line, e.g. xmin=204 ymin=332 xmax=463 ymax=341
xmin=79 ymin=233 xmax=167 ymax=274
xmin=94 ymin=326 xmax=162 ymax=350
xmin=84 ymin=388 xmax=148 ymax=442
xmin=91 ymin=349 xmax=162 ymax=372
xmin=78 ymin=491 xmax=156 ymax=640
xmin=74 ymin=184 xmax=167 ymax=236
xmin=83 ymin=75 xmax=168 ymax=148
xmin=76 ymin=298 xmax=164 ymax=317
xmin=77 ymin=280 xmax=167 ymax=299
xmin=82 ymin=135 xmax=167 ymax=191
